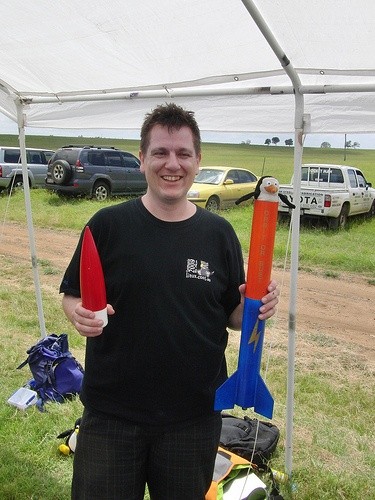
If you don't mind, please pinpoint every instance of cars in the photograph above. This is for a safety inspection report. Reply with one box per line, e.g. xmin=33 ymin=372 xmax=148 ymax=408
xmin=186 ymin=166 xmax=262 ymax=213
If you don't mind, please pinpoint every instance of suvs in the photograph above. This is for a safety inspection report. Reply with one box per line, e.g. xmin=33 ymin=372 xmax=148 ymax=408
xmin=43 ymin=144 xmax=148 ymax=203
xmin=0 ymin=146 xmax=55 ymax=196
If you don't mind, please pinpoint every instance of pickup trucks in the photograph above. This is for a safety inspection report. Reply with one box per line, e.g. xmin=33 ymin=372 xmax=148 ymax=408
xmin=278 ymin=164 xmax=375 ymax=232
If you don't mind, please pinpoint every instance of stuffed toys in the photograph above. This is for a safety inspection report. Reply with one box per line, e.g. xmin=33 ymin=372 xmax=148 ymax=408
xmin=57 ymin=418 xmax=82 ymax=455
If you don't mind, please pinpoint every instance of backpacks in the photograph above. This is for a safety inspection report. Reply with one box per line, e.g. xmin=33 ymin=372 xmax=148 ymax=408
xmin=219 ymin=413 xmax=280 ymax=461
xmin=26 ymin=333 xmax=84 ymax=411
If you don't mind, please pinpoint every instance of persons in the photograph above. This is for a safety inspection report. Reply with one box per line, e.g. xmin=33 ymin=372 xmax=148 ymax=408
xmin=59 ymin=103 xmax=278 ymax=500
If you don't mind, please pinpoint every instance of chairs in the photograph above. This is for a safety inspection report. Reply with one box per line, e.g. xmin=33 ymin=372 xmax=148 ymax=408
xmin=33 ymin=154 xmax=41 ymax=163
xmin=302 ymin=172 xmax=343 ymax=183
